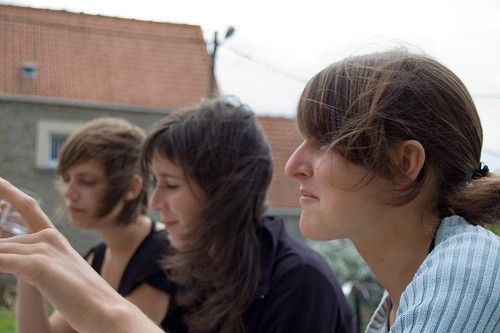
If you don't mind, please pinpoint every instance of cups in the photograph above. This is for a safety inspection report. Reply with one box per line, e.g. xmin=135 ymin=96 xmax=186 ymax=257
xmin=0 ymin=188 xmax=40 ymax=238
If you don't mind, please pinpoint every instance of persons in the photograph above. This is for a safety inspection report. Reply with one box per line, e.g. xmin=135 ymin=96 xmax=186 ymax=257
xmin=0 ymin=118 xmax=172 ymax=333
xmin=0 ymin=45 xmax=497 ymax=333
xmin=140 ymin=93 xmax=355 ymax=333
